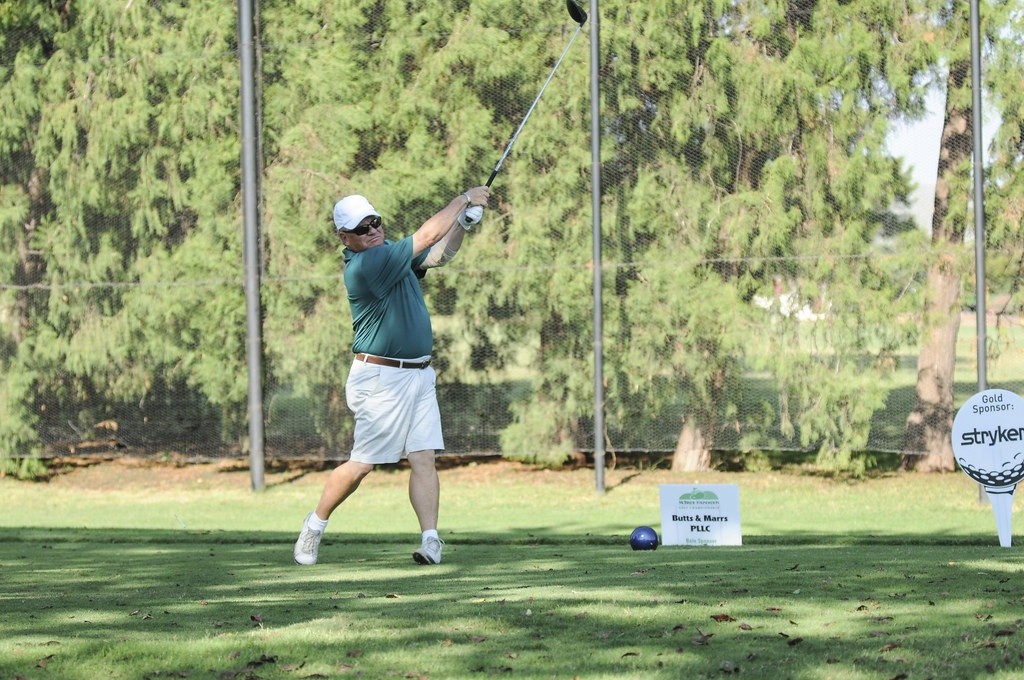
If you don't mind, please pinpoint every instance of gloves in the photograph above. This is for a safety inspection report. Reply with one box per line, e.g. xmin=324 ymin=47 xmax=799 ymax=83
xmin=457 ymin=204 xmax=485 ymax=232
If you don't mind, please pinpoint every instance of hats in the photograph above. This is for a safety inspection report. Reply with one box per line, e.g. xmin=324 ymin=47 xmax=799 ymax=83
xmin=332 ymin=194 xmax=382 ymax=231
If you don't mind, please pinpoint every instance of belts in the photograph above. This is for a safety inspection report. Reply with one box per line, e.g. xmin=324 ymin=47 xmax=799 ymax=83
xmin=355 ymin=352 xmax=431 ymax=369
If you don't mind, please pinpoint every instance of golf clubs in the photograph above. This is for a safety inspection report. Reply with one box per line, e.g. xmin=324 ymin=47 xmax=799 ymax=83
xmin=465 ymin=0 xmax=588 ymax=223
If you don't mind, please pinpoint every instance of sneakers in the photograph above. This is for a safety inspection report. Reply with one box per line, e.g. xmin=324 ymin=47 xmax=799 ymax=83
xmin=292 ymin=511 xmax=325 ymax=566
xmin=412 ymin=535 xmax=446 ymax=565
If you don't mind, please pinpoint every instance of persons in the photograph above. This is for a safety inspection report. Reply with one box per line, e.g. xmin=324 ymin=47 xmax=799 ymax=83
xmin=294 ymin=186 xmax=502 ymax=564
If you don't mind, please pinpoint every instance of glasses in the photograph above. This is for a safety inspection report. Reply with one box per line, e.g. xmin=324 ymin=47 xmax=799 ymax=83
xmin=342 ymin=217 xmax=382 ymax=235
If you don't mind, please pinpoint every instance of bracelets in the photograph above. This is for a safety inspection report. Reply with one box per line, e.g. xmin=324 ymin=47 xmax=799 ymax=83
xmin=462 ymin=192 xmax=471 ymax=207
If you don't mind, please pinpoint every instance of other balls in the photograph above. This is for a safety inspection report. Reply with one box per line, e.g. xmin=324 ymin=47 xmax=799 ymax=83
xmin=630 ymin=526 xmax=659 ymax=550
